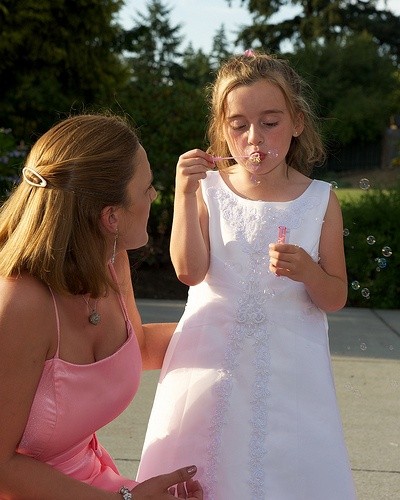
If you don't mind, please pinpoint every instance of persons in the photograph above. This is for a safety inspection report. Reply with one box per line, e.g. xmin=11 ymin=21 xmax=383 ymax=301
xmin=135 ymin=49 xmax=357 ymax=500
xmin=1 ymin=114 xmax=205 ymax=500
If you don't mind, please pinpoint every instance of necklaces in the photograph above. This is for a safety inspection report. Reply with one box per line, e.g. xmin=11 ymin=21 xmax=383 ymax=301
xmin=81 ymin=294 xmax=101 ymax=325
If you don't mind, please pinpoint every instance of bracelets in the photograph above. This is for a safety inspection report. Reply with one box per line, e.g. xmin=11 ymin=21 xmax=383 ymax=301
xmin=118 ymin=485 xmax=135 ymax=500
